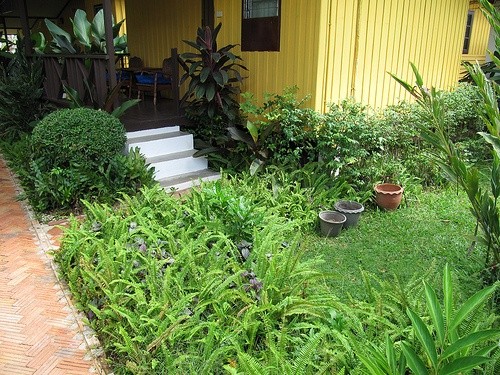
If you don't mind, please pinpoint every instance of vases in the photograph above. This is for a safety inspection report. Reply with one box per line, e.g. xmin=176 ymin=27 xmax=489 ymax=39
xmin=374 ymin=183 xmax=404 ymax=212
xmin=333 ymin=199 xmax=364 ymax=229
xmin=319 ymin=210 xmax=347 ymax=237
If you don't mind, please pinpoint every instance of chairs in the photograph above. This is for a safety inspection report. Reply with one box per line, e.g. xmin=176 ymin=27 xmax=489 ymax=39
xmin=115 ymin=56 xmax=146 ymax=102
xmin=132 ymin=57 xmax=180 ymax=111
